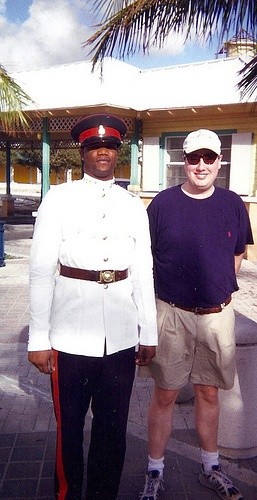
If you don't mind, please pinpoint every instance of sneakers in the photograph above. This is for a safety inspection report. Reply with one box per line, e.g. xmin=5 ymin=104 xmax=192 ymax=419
xmin=198 ymin=462 xmax=243 ymax=500
xmin=139 ymin=466 xmax=165 ymax=500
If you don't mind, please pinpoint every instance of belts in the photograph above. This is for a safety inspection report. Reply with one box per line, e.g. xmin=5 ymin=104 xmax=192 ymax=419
xmin=59 ymin=264 xmax=129 ymax=284
xmin=156 ymin=295 xmax=232 ymax=315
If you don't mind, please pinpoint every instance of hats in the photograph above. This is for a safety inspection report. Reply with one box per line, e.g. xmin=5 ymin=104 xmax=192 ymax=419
xmin=183 ymin=129 xmax=222 ymax=156
xmin=70 ymin=114 xmax=128 ymax=147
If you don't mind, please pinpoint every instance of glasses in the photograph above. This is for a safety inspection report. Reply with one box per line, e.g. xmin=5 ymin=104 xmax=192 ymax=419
xmin=185 ymin=154 xmax=218 ymax=165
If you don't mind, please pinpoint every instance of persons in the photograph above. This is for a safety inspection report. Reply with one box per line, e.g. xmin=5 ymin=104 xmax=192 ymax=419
xmin=137 ymin=129 xmax=253 ymax=500
xmin=27 ymin=114 xmax=158 ymax=500
